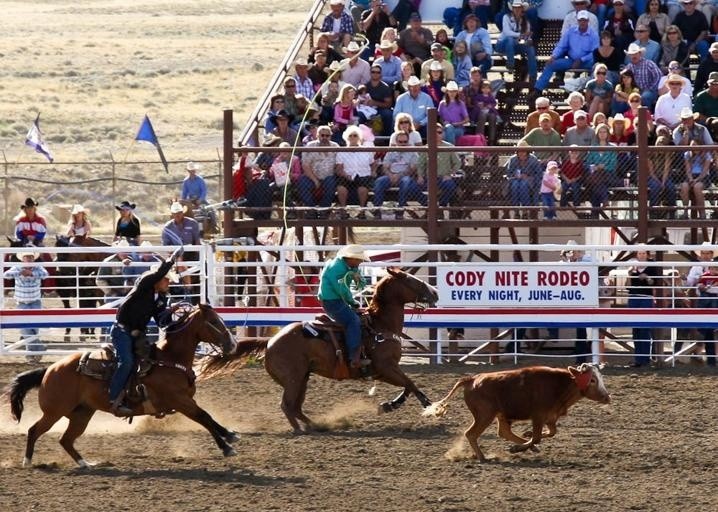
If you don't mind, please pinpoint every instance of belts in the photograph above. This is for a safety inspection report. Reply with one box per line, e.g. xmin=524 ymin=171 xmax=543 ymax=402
xmin=114 ymin=320 xmax=139 ymax=336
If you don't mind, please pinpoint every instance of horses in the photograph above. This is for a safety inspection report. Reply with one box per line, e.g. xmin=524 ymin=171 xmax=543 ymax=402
xmin=4 ymin=235 xmax=46 ymax=300
xmin=197 ymin=267 xmax=449 ymax=433
xmin=50 ymin=233 xmax=97 ymax=340
xmin=66 ymin=228 xmax=167 ymax=341
xmin=4 ymin=304 xmax=250 ymax=448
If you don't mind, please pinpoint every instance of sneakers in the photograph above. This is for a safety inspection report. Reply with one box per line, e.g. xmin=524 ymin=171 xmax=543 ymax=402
xmin=396 ymin=213 xmax=403 ymax=219
xmin=319 ymin=211 xmax=328 ymax=219
xmin=335 ymin=210 xmax=347 ymax=220
xmin=358 ymin=212 xmax=366 ymax=219
xmin=374 ymin=212 xmax=382 ymax=220
xmin=304 ymin=211 xmax=317 ymax=219
xmin=351 ymin=359 xmax=372 ymax=368
xmin=286 ymin=211 xmax=296 ymax=219
xmin=107 ymin=400 xmax=132 ymax=414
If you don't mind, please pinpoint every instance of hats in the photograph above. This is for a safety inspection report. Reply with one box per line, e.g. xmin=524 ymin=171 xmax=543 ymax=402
xmin=507 ymin=0 xmax=529 ymax=11
xmin=16 ymin=252 xmax=40 ymax=261
xmin=306 ymin=102 xmax=319 ymax=111
xmin=513 ymin=141 xmax=529 ymax=153
xmin=573 ymin=110 xmax=586 ymax=121
xmin=323 ymin=60 xmax=346 ymax=73
xmin=425 ymin=61 xmax=447 ymax=73
xmin=337 ymin=243 xmax=371 ymax=262
xmin=167 ymin=201 xmax=188 ymax=214
xmin=623 ymin=42 xmax=646 ymax=56
xmin=676 ymin=106 xmax=700 ymax=120
xmin=112 ymin=240 xmax=129 ymax=247
xmin=613 ymin=0 xmax=625 ymax=4
xmin=185 ymin=162 xmax=196 ymax=171
xmin=375 ymin=39 xmax=398 ymax=53
xmin=431 ymin=42 xmax=441 ymax=50
xmin=547 ymin=161 xmax=557 ymax=169
xmin=570 ymin=0 xmax=591 ymax=6
xmin=567 ymin=240 xmax=578 ymax=246
xmin=21 ymin=198 xmax=38 ymax=209
xmin=441 ymin=81 xmax=463 ymax=93
xmin=344 ymin=41 xmax=363 ymax=52
xmin=290 ymin=58 xmax=312 ymax=71
xmin=115 ymin=201 xmax=135 ymax=211
xmin=693 ymin=241 xmax=718 ymax=258
xmin=539 ymin=113 xmax=552 ymax=122
xmin=664 ymin=74 xmax=685 ymax=89
xmin=330 ymin=0 xmax=345 ymax=6
xmin=262 ymin=132 xmax=282 ymax=146
xmin=140 ymin=241 xmax=152 ymax=246
xmin=608 ymin=112 xmax=631 ymax=135
xmin=150 ymin=262 xmax=179 ymax=284
xmin=316 ymin=49 xmax=327 ymax=56
xmin=270 ymin=108 xmax=294 ymax=124
xmin=706 ymin=72 xmax=718 ymax=84
xmin=709 ymin=41 xmax=718 ymax=51
xmin=576 ymin=9 xmax=589 ymax=20
xmin=678 ymin=0 xmax=703 ymax=6
xmin=402 ymin=75 xmax=424 ymax=91
xmin=68 ymin=204 xmax=90 ymax=215
xmin=305 ymin=118 xmax=322 ymax=131
xmin=409 ymin=12 xmax=420 ymax=19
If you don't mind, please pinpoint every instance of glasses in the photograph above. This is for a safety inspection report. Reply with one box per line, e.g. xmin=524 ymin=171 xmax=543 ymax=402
xmin=286 ymin=85 xmax=295 ymax=88
xmin=636 ymin=30 xmax=648 ymax=33
xmin=668 ymin=31 xmax=677 ymax=34
xmin=371 ymin=71 xmax=380 ymax=74
xmin=631 ymin=100 xmax=638 ymax=102
xmin=538 ymin=107 xmax=546 ymax=109
xmin=349 ymin=134 xmax=357 ymax=137
xmin=437 ymin=131 xmax=442 ymax=134
xmin=321 ymin=133 xmax=331 ymax=137
xmin=597 ymin=71 xmax=606 ymax=75
xmin=669 ymin=82 xmax=681 ymax=85
xmin=684 ymin=1 xmax=692 ymax=5
xmin=399 ymin=140 xmax=407 ymax=143
xmin=400 ymin=120 xmax=409 ymax=124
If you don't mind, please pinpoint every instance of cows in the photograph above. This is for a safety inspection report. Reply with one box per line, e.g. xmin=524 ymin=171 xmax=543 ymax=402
xmin=418 ymin=358 xmax=617 ymax=464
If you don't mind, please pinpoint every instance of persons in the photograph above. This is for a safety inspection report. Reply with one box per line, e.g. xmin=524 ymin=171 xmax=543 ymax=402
xmin=302 ymin=119 xmax=307 ymax=121
xmin=541 ymin=161 xmax=561 ymax=220
xmin=421 ymin=61 xmax=447 ymax=108
xmin=672 ymin=107 xmax=715 ymax=168
xmin=432 ymin=28 xmax=455 ymax=54
xmin=699 ymin=261 xmax=718 ymax=301
xmin=454 ymin=13 xmax=493 ymax=80
xmin=559 ymin=239 xmax=584 ymax=263
xmin=622 ymin=43 xmax=664 ymax=108
xmin=375 ymin=26 xmax=405 ymax=61
xmin=3 ymin=197 xmax=55 ymax=296
xmin=634 ymin=0 xmax=671 ymax=39
xmin=95 ymin=239 xmax=137 ymax=304
xmin=591 ymin=111 xmax=609 ymax=134
xmin=282 ymin=76 xmax=306 ymax=118
xmin=292 ymin=57 xmax=316 ymax=103
xmin=561 ymin=109 xmax=595 ymax=160
xmin=658 ymin=61 xmax=693 ymax=102
xmin=265 ymin=95 xmax=287 ymax=132
xmin=388 ymin=112 xmax=423 ymax=146
xmin=518 ymin=112 xmax=561 ymax=164
xmin=561 ymin=91 xmax=591 ymax=131
xmin=438 ymin=45 xmax=452 ymax=65
xmin=462 ymin=67 xmax=497 ymax=146
xmin=584 ymin=123 xmax=618 ymax=212
xmin=399 ymin=12 xmax=434 ymax=61
xmin=695 ymin=42 xmax=718 ymax=100
xmin=523 ymin=96 xmax=560 ymax=138
xmin=300 ymin=125 xmax=340 ymax=220
xmin=319 ymin=60 xmax=348 ymax=122
xmin=670 ymin=67 xmax=676 ymax=75
xmin=675 ymin=242 xmax=718 ymax=367
xmin=680 ymin=138 xmax=710 ymax=218
xmin=301 ymin=118 xmax=324 ymax=147
xmin=658 ymin=60 xmax=667 ymax=65
xmin=671 ymin=0 xmax=709 ymax=42
xmin=471 ymin=80 xmax=497 ymax=125
xmin=333 ymin=85 xmax=360 ymax=128
xmin=115 ymin=200 xmax=140 ymax=242
xmin=161 ymin=201 xmax=210 ymax=306
xmin=654 ymin=74 xmax=692 ymax=130
xmin=506 ymin=141 xmax=543 ymax=220
xmin=321 ymin=0 xmax=354 ymax=35
xmin=526 ymin=9 xmax=599 ymax=101
xmin=67 ymin=204 xmax=91 ymax=237
xmin=243 ymin=132 xmax=284 ymax=219
xmin=181 ymin=161 xmax=218 ymax=226
xmin=624 ymin=24 xmax=661 ymax=65
xmin=339 ymin=42 xmax=371 ymax=89
xmin=326 ymin=81 xmax=339 ymax=110
xmin=662 ymin=106 xmax=664 ymax=106
xmin=608 ymin=113 xmax=632 ymax=161
xmin=2 ymin=245 xmax=50 ymax=366
xmin=317 ymin=244 xmax=369 ymax=371
xmin=417 ymin=123 xmax=462 ymax=220
xmin=560 ymin=0 xmax=599 ymax=38
xmin=438 ymin=80 xmax=470 ymax=145
xmin=269 ymin=142 xmax=301 ymax=220
xmin=623 ymin=92 xmax=654 ymax=142
xmin=393 ymin=61 xmax=418 ymax=101
xmin=307 ymin=34 xmax=346 ymax=67
xmin=584 ymin=62 xmax=613 ymax=121
xmin=359 ymin=0 xmax=396 ymax=44
xmin=614 ymin=69 xmax=640 ymax=114
xmin=560 ymin=144 xmax=585 ymax=209
xmin=420 ymin=43 xmax=455 ymax=82
xmin=659 ymin=25 xmax=688 ymax=68
xmin=392 ymin=76 xmax=434 ymax=138
xmin=593 ymin=31 xmax=620 ymax=87
xmin=265 ymin=94 xmax=269 ymax=99
xmin=307 ymin=52 xmax=328 ymax=105
xmin=692 ymin=71 xmax=718 ymax=123
xmin=122 ymin=240 xmax=165 ymax=298
xmin=371 ymin=39 xmax=403 ymax=85
xmin=301 ymin=107 xmax=321 ymax=137
xmin=269 ymin=109 xmax=302 ymax=146
xmin=363 ymin=65 xmax=393 ymax=129
xmin=374 ymin=132 xmax=418 ymax=220
xmin=495 ymin=0 xmax=538 ymax=86
xmin=336 ymin=125 xmax=375 ymax=219
xmin=602 ymin=0 xmax=637 ymax=40
xmin=624 ymin=251 xmax=657 ymax=368
xmin=647 ymin=136 xmax=676 ymax=219
xmin=109 ymin=245 xmax=184 ymax=415
xmin=356 ymin=85 xmax=379 ymax=118
